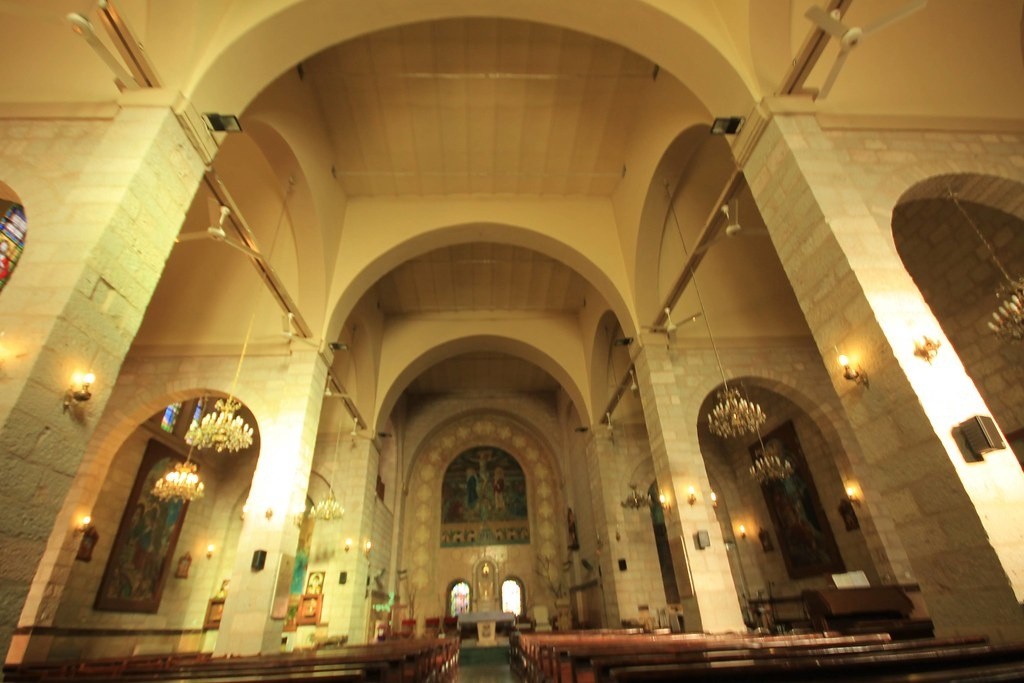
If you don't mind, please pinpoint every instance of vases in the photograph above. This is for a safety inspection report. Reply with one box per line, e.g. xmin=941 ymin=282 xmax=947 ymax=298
xmin=517 ymin=624 xmax=530 ymax=629
xmin=403 ymin=623 xmax=458 ymax=637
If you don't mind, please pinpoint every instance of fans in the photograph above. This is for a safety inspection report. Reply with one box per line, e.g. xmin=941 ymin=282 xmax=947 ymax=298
xmin=593 ymin=0 xmax=931 ymax=464
xmin=58 ymin=12 xmax=383 ymax=450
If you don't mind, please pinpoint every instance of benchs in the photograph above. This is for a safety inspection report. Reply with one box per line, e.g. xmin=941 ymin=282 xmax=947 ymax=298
xmin=508 ymin=630 xmax=1024 ymax=683
xmin=0 ymin=637 xmax=465 ymax=683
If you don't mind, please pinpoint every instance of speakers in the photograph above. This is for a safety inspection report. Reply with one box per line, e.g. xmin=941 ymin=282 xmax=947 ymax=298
xmin=619 ymin=560 xmax=626 ymax=570
xmin=252 ymin=550 xmax=266 ymax=570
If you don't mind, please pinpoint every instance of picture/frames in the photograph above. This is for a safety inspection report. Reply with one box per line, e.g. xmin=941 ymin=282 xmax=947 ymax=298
xmin=841 ymin=501 xmax=858 ymax=531
xmin=93 ymin=438 xmax=200 ymax=614
xmin=748 ymin=421 xmax=844 ymax=578
xmin=75 ymin=528 xmax=98 ymax=559
xmin=174 ymin=552 xmax=195 ymax=579
xmin=758 ymin=527 xmax=774 ymax=551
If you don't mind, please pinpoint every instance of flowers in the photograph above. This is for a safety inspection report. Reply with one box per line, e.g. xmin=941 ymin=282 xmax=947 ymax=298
xmin=402 ymin=615 xmax=459 ymax=626
xmin=517 ymin=615 xmax=531 ymax=624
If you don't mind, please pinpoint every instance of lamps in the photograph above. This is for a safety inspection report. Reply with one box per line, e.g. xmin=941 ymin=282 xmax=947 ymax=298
xmin=206 ymin=110 xmax=243 ymax=135
xmin=617 ymin=117 xmax=794 ymax=583
xmin=150 ymin=325 xmax=373 ymax=585
xmin=73 ymin=515 xmax=98 ymax=538
xmin=833 ymin=175 xmax=1023 ymax=506
xmin=65 ymin=366 xmax=94 ymax=409
